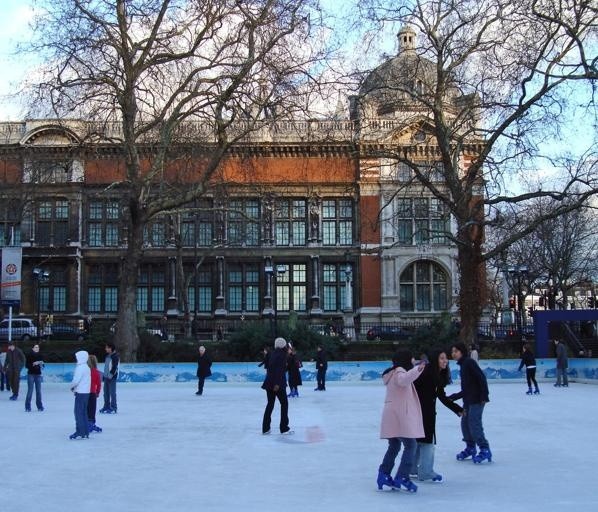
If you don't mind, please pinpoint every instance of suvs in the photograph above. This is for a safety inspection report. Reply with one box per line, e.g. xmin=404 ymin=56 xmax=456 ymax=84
xmin=0 ymin=317 xmax=45 ymax=342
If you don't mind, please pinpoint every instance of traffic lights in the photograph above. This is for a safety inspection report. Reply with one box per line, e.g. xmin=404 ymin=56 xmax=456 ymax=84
xmin=527 ymin=307 xmax=532 ymax=318
xmin=586 ymin=295 xmax=593 ymax=308
xmin=508 ymin=299 xmax=514 ymax=309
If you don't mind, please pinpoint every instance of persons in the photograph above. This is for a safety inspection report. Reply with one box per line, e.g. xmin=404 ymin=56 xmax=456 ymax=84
xmin=88 ymin=355 xmax=102 ymax=431
xmin=411 ymin=352 xmax=430 ymax=364
xmin=551 ymin=337 xmax=571 ymax=385
xmin=25 ymin=345 xmax=46 ymax=414
xmin=446 ymin=344 xmax=492 ymax=462
xmin=411 ymin=351 xmax=467 ymax=480
xmin=261 ymin=336 xmax=296 ymax=435
xmin=376 ymin=350 xmax=427 ymax=492
xmin=99 ymin=343 xmax=120 ymax=414
xmin=518 ymin=344 xmax=539 ymax=393
xmin=0 ymin=342 xmax=25 ymax=400
xmin=314 ymin=345 xmax=329 ymax=392
xmin=195 ymin=345 xmax=214 ymax=396
xmin=67 ymin=350 xmax=90 ymax=439
xmin=468 ymin=345 xmax=481 ymax=365
xmin=287 ymin=342 xmax=306 ymax=396
xmin=257 ymin=345 xmax=271 ymax=372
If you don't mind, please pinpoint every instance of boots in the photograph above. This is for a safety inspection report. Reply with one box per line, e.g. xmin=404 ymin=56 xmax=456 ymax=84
xmin=455 ymin=444 xmax=478 ymax=458
xmin=9 ymin=394 xmax=44 ymax=411
xmin=393 ymin=474 xmax=418 ymax=490
xmin=69 ymin=423 xmax=102 ymax=438
xmin=526 ymin=388 xmax=540 ymax=393
xmin=286 ymin=386 xmax=327 ymax=397
xmin=472 ymin=447 xmax=494 ymax=462
xmin=377 ymin=472 xmax=400 ymax=488
xmin=553 ymin=381 xmax=568 ymax=386
xmin=99 ymin=406 xmax=117 ymax=413
xmin=418 ymin=471 xmax=442 ymax=481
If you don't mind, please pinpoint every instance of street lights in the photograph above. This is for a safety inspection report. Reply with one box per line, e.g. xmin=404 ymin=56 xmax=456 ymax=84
xmin=31 ymin=267 xmax=51 ymax=347
xmin=264 ymin=265 xmax=286 ymax=338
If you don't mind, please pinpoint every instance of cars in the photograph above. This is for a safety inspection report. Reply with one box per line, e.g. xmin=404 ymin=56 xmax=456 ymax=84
xmin=365 ymin=325 xmax=413 ymax=342
xmin=308 ymin=323 xmax=351 ymax=344
xmin=480 ymin=324 xmax=534 ymax=339
xmin=42 ymin=322 xmax=87 ymax=343
xmin=110 ymin=321 xmax=163 ymax=338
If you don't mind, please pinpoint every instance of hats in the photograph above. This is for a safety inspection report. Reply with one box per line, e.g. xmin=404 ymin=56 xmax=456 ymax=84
xmin=391 ymin=346 xmax=416 ymax=367
xmin=273 ymin=337 xmax=287 ymax=349
xmin=450 ymin=341 xmax=468 ymax=355
